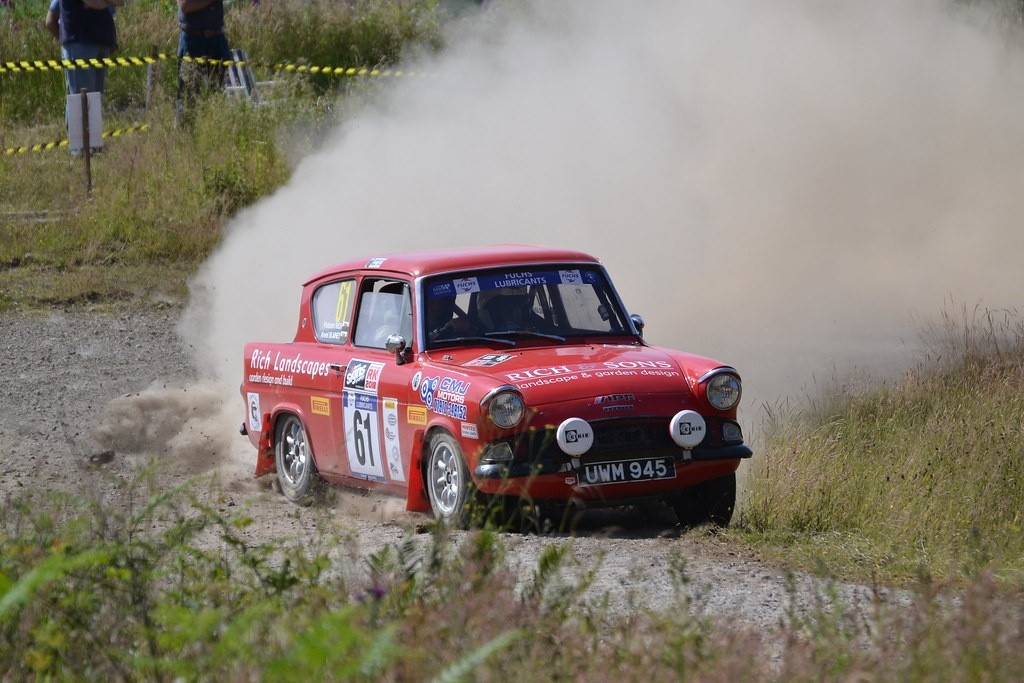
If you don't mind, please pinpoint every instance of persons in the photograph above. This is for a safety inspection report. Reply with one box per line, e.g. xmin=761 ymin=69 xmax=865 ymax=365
xmin=172 ymin=0 xmax=229 ymax=133
xmin=45 ymin=0 xmax=125 ymax=157
xmin=476 ymin=288 xmax=554 ymax=332
xmin=374 ymin=279 xmax=476 ymax=351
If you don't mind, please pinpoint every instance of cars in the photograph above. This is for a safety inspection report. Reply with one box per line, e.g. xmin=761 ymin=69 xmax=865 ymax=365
xmin=236 ymin=247 xmax=755 ymax=531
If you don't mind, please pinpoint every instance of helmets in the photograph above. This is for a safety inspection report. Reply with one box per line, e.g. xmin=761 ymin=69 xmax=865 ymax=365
xmin=482 ymin=285 xmax=527 ymax=312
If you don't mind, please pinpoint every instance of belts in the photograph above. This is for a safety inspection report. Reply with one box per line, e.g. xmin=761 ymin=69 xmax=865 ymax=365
xmin=180 ymin=25 xmax=225 ymax=38
